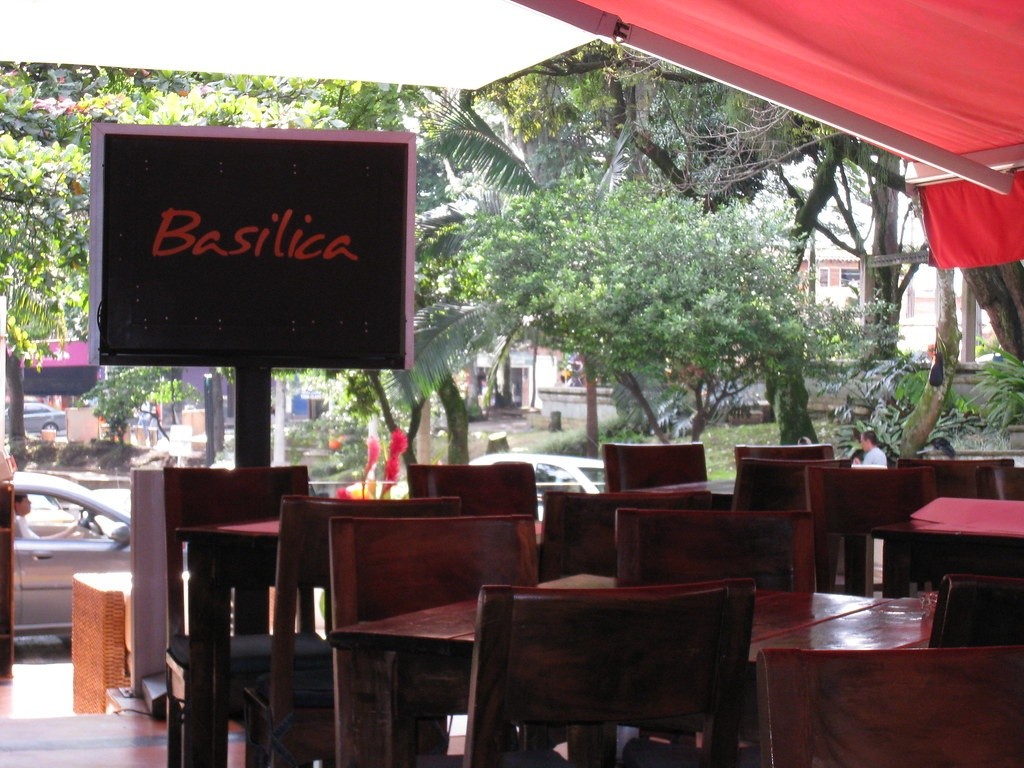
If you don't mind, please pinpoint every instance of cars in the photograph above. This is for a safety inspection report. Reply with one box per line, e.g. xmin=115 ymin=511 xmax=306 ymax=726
xmin=5 ymin=402 xmax=66 ymax=438
xmin=12 ymin=469 xmax=130 ymax=645
xmin=465 ymin=453 xmax=607 ymax=520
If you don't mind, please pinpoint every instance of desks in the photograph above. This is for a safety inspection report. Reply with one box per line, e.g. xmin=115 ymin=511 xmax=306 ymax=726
xmin=870 ymin=496 xmax=1024 ymax=598
xmin=175 ymin=520 xmax=281 ymax=768
xmin=621 ymin=477 xmax=875 ymax=596
xmin=327 ymin=572 xmax=939 ymax=768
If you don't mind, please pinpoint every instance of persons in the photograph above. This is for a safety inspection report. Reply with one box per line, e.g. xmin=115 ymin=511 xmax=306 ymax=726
xmin=852 ymin=429 xmax=887 ymax=467
xmin=13 ymin=495 xmax=79 ymax=540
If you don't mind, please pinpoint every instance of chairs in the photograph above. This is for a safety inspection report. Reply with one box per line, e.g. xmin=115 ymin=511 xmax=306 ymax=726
xmin=71 ymin=442 xmax=1024 ymax=768
